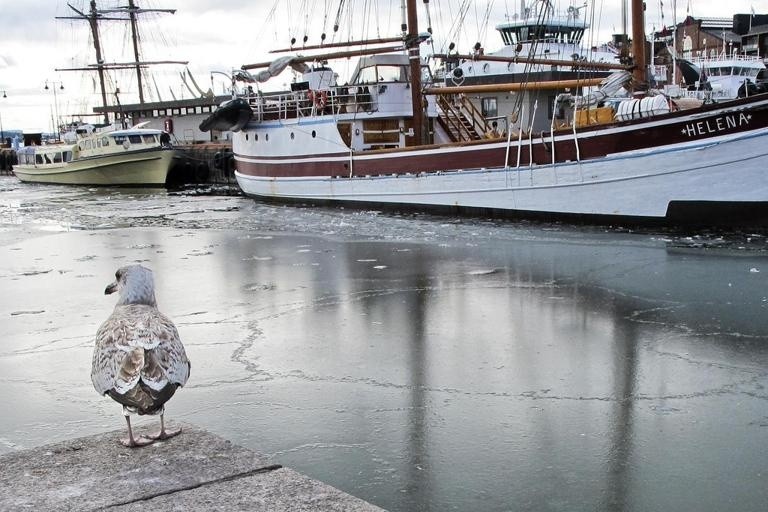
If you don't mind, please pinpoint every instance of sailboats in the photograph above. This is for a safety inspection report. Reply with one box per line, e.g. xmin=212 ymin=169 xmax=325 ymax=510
xmin=204 ymin=0 xmax=768 ymax=237
xmin=10 ymin=0 xmax=234 ymax=191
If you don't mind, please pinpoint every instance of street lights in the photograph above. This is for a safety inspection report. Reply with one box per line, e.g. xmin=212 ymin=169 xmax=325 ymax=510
xmin=44 ymin=79 xmax=65 ymax=141
xmin=0 ymin=90 xmax=7 ymax=147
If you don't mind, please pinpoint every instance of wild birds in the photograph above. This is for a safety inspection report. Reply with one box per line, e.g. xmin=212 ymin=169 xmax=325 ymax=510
xmin=90 ymin=264 xmax=190 ymax=449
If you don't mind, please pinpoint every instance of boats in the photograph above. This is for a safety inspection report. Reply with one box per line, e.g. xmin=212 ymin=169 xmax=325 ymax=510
xmin=645 ymin=1 xmax=766 ymax=100
xmin=10 ymin=127 xmax=230 ymax=186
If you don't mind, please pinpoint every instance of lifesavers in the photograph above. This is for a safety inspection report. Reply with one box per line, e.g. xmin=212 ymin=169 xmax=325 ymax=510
xmin=214 ymin=152 xmax=224 ymax=170
xmin=314 ymin=92 xmax=327 ymax=110
